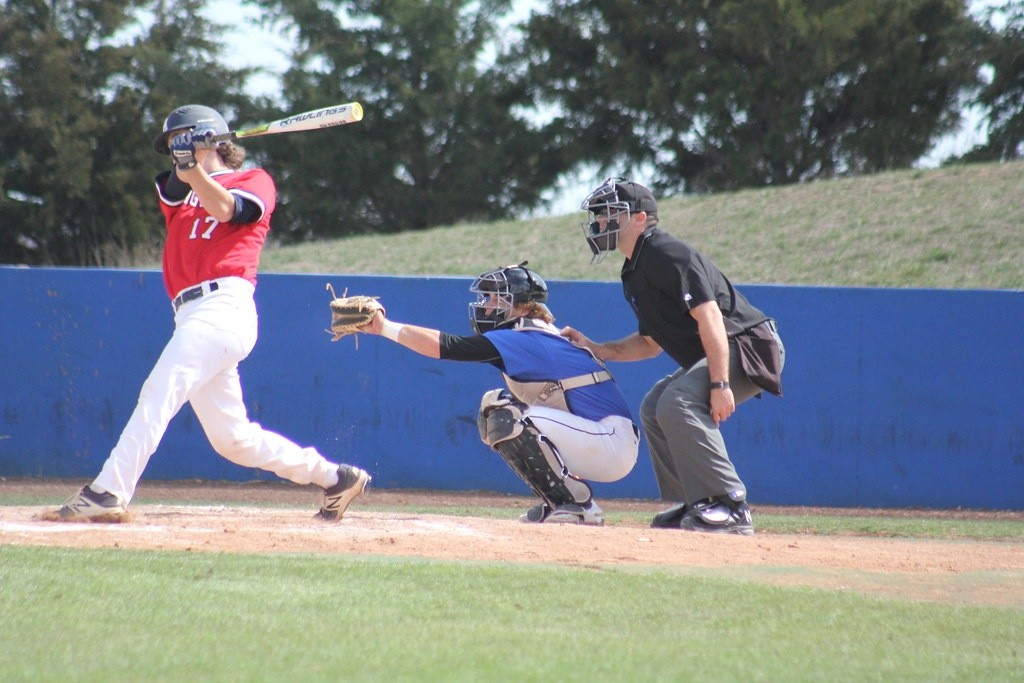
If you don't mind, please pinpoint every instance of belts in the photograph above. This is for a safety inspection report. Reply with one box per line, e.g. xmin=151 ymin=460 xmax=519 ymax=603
xmin=175 ymin=282 xmax=219 ymax=313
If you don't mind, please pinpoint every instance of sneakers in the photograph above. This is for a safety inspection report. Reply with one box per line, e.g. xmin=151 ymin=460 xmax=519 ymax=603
xmin=544 ymin=503 xmax=605 ymax=525
xmin=311 ymin=462 xmax=371 ymax=521
xmin=651 ymin=497 xmax=710 ymax=531
xmin=680 ymin=496 xmax=754 ymax=535
xmin=41 ymin=483 xmax=121 ymax=523
xmin=517 ymin=504 xmax=550 ymax=524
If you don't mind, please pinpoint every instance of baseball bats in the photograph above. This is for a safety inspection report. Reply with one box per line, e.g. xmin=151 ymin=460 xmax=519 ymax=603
xmin=212 ymin=101 xmax=364 ymax=143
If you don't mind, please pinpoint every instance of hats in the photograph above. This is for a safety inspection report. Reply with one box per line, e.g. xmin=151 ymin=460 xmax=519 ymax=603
xmin=589 ymin=180 xmax=659 ymax=212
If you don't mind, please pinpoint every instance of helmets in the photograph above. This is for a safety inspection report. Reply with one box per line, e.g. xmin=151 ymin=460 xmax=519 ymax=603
xmin=478 ymin=259 xmax=548 ymax=304
xmin=154 ymin=104 xmax=230 ymax=155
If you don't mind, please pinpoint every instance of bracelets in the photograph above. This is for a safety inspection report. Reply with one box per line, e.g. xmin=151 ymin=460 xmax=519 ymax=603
xmin=710 ymin=382 xmax=730 ymax=389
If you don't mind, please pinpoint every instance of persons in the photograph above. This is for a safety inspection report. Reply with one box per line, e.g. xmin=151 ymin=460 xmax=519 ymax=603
xmin=329 ymin=261 xmax=640 ymax=526
xmin=40 ymin=104 xmax=373 ymax=526
xmin=559 ymin=176 xmax=786 ymax=534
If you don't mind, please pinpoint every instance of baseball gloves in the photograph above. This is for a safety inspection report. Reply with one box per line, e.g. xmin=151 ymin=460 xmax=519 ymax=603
xmin=324 ymin=283 xmax=387 ymax=352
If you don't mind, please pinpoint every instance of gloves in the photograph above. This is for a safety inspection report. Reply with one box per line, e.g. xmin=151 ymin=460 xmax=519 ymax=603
xmin=191 ymin=126 xmax=219 ymax=150
xmin=170 ymin=127 xmax=197 ymax=170
